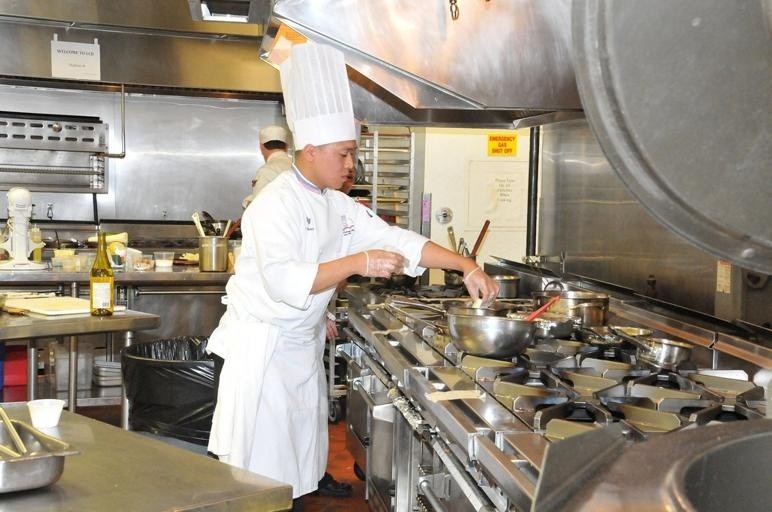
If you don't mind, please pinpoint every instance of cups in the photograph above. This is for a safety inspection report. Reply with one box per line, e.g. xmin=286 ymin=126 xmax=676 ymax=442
xmin=78 ymin=248 xmax=97 ymax=272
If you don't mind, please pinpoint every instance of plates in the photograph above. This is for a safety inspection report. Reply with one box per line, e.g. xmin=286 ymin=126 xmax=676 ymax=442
xmin=1 ymin=419 xmax=78 ymax=495
xmin=93 ymin=360 xmax=123 ymax=387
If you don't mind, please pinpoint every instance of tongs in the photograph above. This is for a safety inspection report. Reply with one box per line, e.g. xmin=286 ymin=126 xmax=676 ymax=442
xmin=385 ymin=297 xmax=448 ymax=335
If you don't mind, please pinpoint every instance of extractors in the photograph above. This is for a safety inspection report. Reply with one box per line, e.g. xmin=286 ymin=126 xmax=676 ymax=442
xmin=178 ymin=0 xmax=275 ymax=37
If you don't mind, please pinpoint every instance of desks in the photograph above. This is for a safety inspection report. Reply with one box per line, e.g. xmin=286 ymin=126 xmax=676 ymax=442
xmin=0 ymin=291 xmax=159 ymax=430
xmin=0 ymin=397 xmax=295 ymax=512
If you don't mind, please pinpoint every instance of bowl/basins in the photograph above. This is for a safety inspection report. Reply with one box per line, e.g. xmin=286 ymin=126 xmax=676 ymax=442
xmin=52 ymin=257 xmax=76 ymax=271
xmin=444 ymin=308 xmax=539 ymax=359
xmin=153 ymin=252 xmax=175 ymax=267
xmin=27 ymin=399 xmax=65 ymax=429
xmin=489 ymin=274 xmax=521 ymax=299
xmin=530 ymin=290 xmax=609 ymax=317
xmin=616 ymin=325 xmax=652 ymax=338
xmin=646 ymin=338 xmax=693 ymax=368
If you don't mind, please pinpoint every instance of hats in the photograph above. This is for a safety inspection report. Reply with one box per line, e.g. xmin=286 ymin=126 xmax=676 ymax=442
xmin=279 ymin=41 xmax=358 ymax=152
xmin=259 ymin=125 xmax=288 ymax=144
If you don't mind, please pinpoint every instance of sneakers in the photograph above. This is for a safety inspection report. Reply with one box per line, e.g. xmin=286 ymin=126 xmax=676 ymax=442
xmin=310 ymin=473 xmax=353 ymax=496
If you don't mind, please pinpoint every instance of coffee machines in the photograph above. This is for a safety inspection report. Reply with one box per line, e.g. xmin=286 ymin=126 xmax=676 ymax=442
xmin=6 ymin=186 xmax=33 ymax=268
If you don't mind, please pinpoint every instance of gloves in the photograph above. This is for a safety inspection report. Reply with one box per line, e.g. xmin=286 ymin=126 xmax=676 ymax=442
xmin=362 ymin=247 xmax=407 ymax=280
xmin=462 ymin=265 xmax=501 ymax=308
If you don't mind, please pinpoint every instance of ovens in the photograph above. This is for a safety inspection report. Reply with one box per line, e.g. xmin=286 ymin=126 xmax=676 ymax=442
xmin=0 ymin=110 xmax=111 ymax=194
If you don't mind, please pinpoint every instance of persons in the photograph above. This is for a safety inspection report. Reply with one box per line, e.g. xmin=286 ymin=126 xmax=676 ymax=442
xmin=205 ymin=45 xmax=500 ymax=512
xmin=327 ymin=118 xmax=363 ymax=340
xmin=241 ymin=126 xmax=293 ymax=209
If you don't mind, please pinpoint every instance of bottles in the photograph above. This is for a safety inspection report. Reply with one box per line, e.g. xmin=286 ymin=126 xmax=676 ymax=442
xmin=89 ymin=231 xmax=115 ymax=316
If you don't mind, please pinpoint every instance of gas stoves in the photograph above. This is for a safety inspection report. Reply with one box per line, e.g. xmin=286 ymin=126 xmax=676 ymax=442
xmin=338 ymin=281 xmax=770 ymax=469
